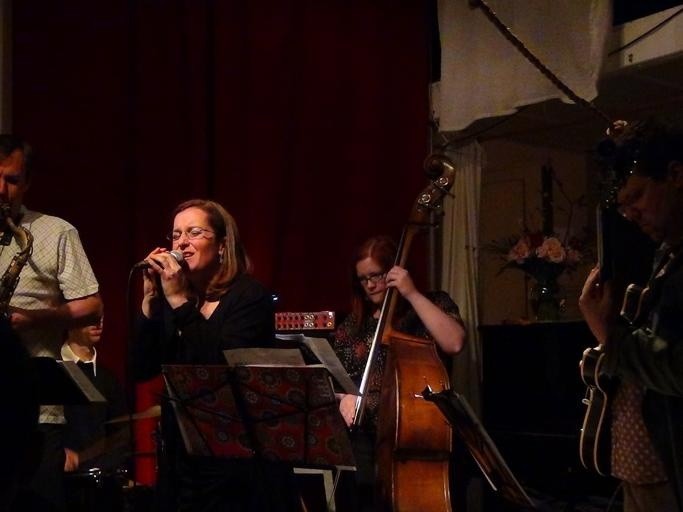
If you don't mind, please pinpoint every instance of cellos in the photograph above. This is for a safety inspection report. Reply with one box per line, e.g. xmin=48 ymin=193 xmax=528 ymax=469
xmin=367 ymin=153 xmax=456 ymax=511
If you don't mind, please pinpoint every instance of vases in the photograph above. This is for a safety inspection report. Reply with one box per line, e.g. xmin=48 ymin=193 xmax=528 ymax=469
xmin=530 ymin=279 xmax=568 ymax=321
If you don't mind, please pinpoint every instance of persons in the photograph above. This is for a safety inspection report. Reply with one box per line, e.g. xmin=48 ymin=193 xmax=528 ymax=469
xmin=123 ymin=198 xmax=276 ymax=511
xmin=0 ymin=134 xmax=103 ymax=511
xmin=577 ymin=110 xmax=683 ymax=512
xmin=58 ymin=308 xmax=139 ymax=511
xmin=330 ymin=234 xmax=468 ymax=512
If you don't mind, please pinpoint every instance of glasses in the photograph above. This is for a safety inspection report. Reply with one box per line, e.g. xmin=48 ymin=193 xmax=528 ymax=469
xmin=353 ymin=268 xmax=388 ymax=289
xmin=165 ymin=227 xmax=219 ymax=241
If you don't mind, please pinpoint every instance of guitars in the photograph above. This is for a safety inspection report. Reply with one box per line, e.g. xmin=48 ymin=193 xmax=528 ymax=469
xmin=581 ymin=121 xmax=642 ymax=479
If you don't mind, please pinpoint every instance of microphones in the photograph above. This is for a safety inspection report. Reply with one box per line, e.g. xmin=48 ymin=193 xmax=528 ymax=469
xmin=133 ymin=249 xmax=186 ymax=269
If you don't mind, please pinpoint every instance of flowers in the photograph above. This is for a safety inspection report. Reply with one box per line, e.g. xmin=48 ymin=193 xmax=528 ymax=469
xmin=478 ymin=227 xmax=586 ymax=279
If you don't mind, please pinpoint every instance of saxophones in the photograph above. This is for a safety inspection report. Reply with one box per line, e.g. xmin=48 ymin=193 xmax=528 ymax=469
xmin=0 ymin=206 xmax=33 ymax=321
